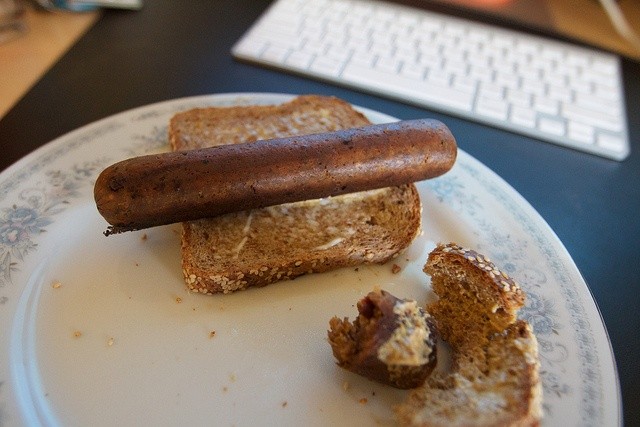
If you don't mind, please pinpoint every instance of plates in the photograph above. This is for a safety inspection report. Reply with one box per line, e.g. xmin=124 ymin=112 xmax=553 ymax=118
xmin=1 ymin=94 xmax=624 ymax=427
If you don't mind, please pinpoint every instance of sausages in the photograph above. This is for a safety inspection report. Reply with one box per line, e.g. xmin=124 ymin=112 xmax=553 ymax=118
xmin=94 ymin=117 xmax=458 ymax=237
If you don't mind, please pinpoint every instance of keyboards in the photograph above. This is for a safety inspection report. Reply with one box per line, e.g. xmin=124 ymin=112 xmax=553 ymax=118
xmin=231 ymin=0 xmax=630 ymax=163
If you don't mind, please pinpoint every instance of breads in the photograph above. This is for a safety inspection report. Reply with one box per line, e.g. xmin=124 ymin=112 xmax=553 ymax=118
xmin=168 ymin=95 xmax=421 ymax=296
xmin=327 ymin=288 xmax=438 ymax=391
xmin=388 ymin=241 xmax=543 ymax=426
